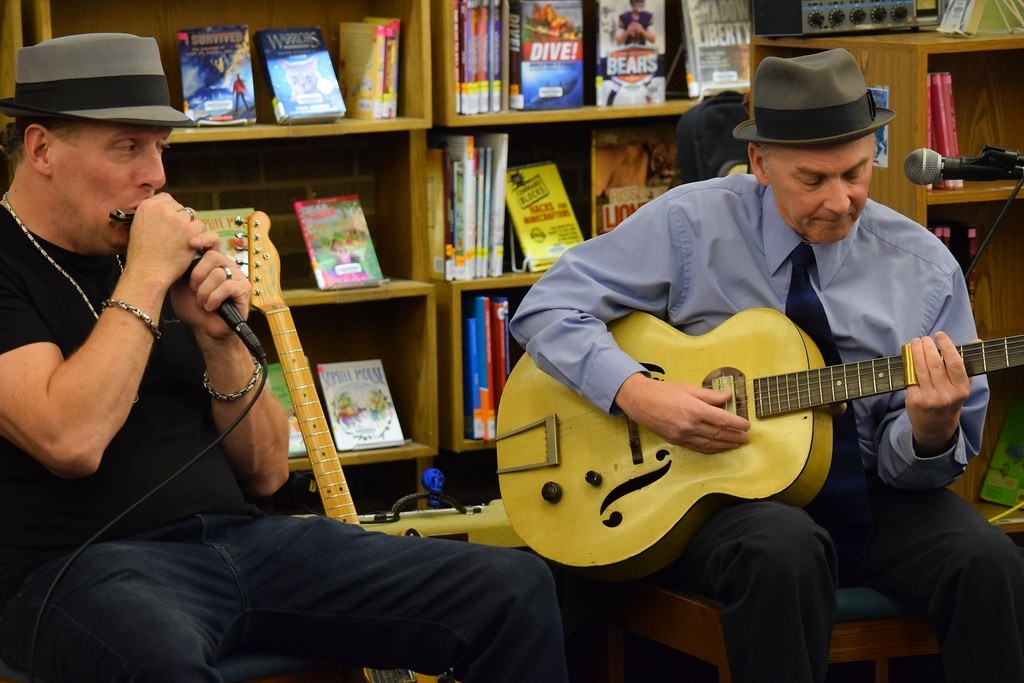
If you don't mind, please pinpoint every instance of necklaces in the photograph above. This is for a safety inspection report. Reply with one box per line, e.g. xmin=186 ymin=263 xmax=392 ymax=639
xmin=7 ymin=197 xmax=138 ymax=404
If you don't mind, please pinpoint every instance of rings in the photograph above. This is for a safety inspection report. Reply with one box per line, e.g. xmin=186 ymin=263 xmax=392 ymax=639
xmin=183 ymin=208 xmax=194 ymax=221
xmin=220 ymin=266 xmax=232 ymax=280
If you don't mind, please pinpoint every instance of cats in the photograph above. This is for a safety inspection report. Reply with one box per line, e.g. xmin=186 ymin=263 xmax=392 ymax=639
xmin=277 ymin=54 xmax=333 ymax=101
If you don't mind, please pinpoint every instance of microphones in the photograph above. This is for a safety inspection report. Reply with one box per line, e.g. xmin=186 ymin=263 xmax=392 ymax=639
xmin=186 ymin=250 xmax=267 ymax=362
xmin=903 ymin=148 xmax=1024 ymax=185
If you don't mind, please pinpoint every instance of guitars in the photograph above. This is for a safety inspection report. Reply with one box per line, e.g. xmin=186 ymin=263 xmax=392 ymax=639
xmin=493 ymin=310 xmax=1024 ymax=583
xmin=235 ymin=210 xmax=462 ymax=683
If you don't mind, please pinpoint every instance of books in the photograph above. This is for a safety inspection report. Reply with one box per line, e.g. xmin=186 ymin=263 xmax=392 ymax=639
xmin=177 ymin=23 xmax=257 ymax=128
xmin=265 ymin=362 xmax=308 ymax=458
xmin=980 ymin=393 xmax=1024 ymax=512
xmin=294 ymin=193 xmax=383 ymax=291
xmin=591 ymin=124 xmax=680 ymax=238
xmin=508 ymin=0 xmax=753 ymax=110
xmin=928 ymin=216 xmax=976 ymax=318
xmin=425 ymin=133 xmax=508 ymax=281
xmin=253 ymin=26 xmax=346 ymax=125
xmin=463 ymin=295 xmax=514 ymax=442
xmin=318 ymin=358 xmax=405 ymax=452
xmin=338 ymin=16 xmax=401 ymax=121
xmin=926 ymin=69 xmax=963 ymax=192
xmin=453 ymin=0 xmax=501 ymax=115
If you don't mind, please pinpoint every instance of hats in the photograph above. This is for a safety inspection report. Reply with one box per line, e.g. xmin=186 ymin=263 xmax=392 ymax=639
xmin=732 ymin=47 xmax=896 ymax=144
xmin=0 ymin=32 xmax=196 ymax=128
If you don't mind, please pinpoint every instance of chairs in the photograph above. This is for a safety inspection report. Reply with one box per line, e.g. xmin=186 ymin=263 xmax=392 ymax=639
xmin=599 ymin=564 xmax=943 ymax=683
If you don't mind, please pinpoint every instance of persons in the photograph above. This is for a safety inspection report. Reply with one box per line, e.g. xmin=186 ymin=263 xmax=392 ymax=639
xmin=511 ymin=45 xmax=1024 ymax=683
xmin=0 ymin=32 xmax=566 ymax=683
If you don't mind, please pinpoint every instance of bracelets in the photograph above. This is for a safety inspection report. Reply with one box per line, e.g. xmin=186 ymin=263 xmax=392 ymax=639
xmin=901 ymin=345 xmax=919 ymax=389
xmin=102 ymin=300 xmax=161 ymax=339
xmin=204 ymin=356 xmax=262 ymax=401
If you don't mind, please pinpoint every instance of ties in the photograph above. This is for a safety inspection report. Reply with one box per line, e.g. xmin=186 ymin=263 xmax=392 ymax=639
xmin=782 ymin=240 xmax=886 ymax=623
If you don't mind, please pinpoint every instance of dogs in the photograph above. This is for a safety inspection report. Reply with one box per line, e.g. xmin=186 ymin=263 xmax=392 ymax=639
xmin=595 ymin=136 xmax=678 ymax=198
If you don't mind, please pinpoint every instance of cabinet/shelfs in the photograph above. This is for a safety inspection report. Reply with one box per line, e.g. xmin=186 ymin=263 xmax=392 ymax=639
xmin=750 ymin=32 xmax=1024 ymax=553
xmin=0 ymin=0 xmax=749 ymax=511
